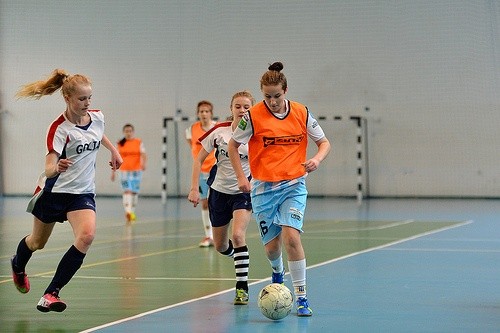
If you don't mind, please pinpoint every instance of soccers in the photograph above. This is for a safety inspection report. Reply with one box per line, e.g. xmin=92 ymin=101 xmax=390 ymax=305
xmin=258 ymin=284 xmax=294 ymax=320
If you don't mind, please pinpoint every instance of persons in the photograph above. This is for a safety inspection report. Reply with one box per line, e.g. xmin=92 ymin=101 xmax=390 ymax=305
xmin=227 ymin=61 xmax=332 ymax=317
xmin=188 ymin=90 xmax=257 ymax=307
xmin=112 ymin=123 xmax=148 ymax=222
xmin=10 ymin=67 xmax=124 ymax=313
xmin=186 ymin=100 xmax=223 ymax=248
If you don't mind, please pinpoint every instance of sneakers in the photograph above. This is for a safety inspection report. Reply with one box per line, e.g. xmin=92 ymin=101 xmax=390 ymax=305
xmin=296 ymin=296 xmax=312 ymax=317
xmin=199 ymin=236 xmax=214 ymax=247
xmin=234 ymin=285 xmax=249 ymax=305
xmin=271 ymin=267 xmax=286 ymax=286
xmin=37 ymin=290 xmax=67 ymax=312
xmin=10 ymin=255 xmax=31 ymax=294
xmin=131 ymin=212 xmax=136 ymax=221
xmin=126 ymin=212 xmax=131 ymax=221
